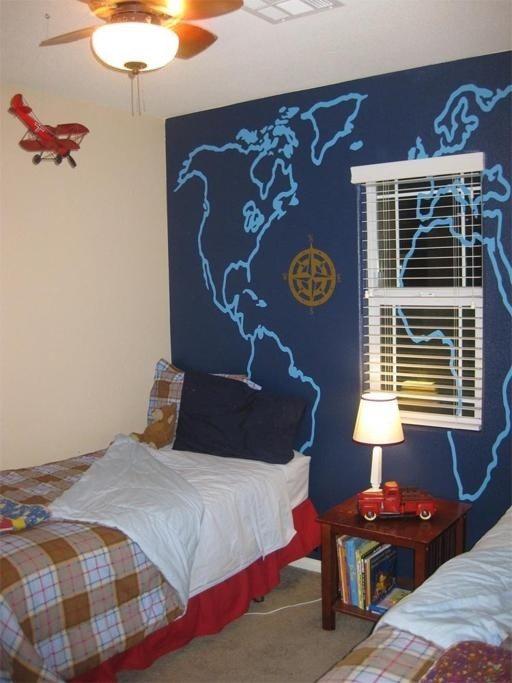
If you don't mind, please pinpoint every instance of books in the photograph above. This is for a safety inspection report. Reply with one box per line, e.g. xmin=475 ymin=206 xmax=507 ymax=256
xmin=336 ymin=534 xmax=414 ymax=617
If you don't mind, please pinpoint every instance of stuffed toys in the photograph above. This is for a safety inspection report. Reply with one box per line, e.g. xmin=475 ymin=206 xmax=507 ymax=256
xmin=129 ymin=403 xmax=177 ymax=451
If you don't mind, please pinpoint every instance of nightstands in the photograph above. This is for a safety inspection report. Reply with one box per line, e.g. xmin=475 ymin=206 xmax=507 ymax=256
xmin=314 ymin=492 xmax=472 ymax=630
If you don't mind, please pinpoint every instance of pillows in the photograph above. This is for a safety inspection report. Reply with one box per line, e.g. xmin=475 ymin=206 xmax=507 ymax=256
xmin=141 ymin=357 xmax=263 ymax=449
xmin=172 ymin=370 xmax=308 ymax=464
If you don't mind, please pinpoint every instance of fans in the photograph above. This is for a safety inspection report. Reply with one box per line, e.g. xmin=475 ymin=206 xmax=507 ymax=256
xmin=38 ymin=1 xmax=243 ymax=58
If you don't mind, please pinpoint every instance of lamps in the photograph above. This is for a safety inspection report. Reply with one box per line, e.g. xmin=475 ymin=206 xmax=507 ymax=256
xmin=90 ymin=21 xmax=180 ymax=115
xmin=352 ymin=391 xmax=405 ymax=492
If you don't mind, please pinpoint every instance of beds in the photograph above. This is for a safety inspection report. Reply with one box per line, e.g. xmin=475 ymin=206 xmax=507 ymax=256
xmin=0 ymin=437 xmax=321 ymax=682
xmin=315 ymin=501 xmax=512 ymax=683
